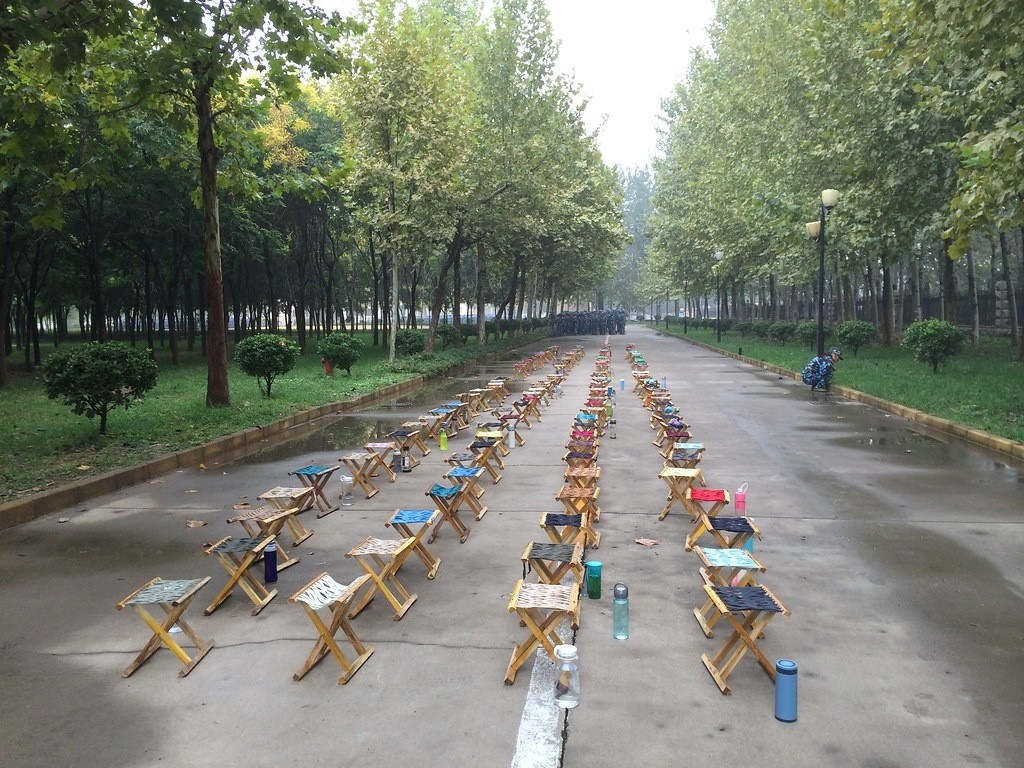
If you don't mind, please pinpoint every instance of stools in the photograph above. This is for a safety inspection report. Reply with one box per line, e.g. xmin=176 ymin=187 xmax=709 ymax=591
xmin=685 ymin=488 xmax=730 ymax=552
xmin=385 ymin=508 xmax=442 ymax=580
xmin=537 ymin=512 xmax=586 ymax=588
xmin=519 ymin=541 xmax=583 ymax=632
xmin=425 ymin=482 xmax=470 ymax=543
xmin=257 ymin=486 xmax=314 ymax=547
xmin=699 ymin=514 xmax=760 ymax=588
xmin=693 ymin=545 xmax=767 ymax=641
xmin=701 ymin=585 xmax=791 ymax=696
xmin=337 ymin=346 xmax=706 ymax=521
xmin=288 ymin=571 xmax=375 ymax=685
xmin=204 ymin=535 xmax=279 ymax=617
xmin=503 ymin=579 xmax=579 ymax=690
xmin=345 ymin=536 xmax=419 ymax=621
xmin=117 ymin=576 xmax=214 ymax=678
xmin=288 ymin=464 xmax=341 ymax=518
xmin=226 ymin=506 xmax=300 ymax=572
xmin=555 ymin=486 xmax=601 ymax=548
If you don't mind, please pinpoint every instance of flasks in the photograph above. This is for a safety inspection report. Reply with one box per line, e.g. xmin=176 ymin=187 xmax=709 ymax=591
xmin=775 ymin=659 xmax=797 ymax=723
xmin=508 ymin=425 xmax=515 ymax=448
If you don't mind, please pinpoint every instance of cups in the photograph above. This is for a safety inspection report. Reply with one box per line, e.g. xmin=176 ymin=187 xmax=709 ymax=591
xmin=608 ymin=386 xmax=612 ymax=398
xmin=610 ymin=420 xmax=616 ymax=439
xmin=606 ymin=404 xmax=612 ymax=418
xmin=585 ymin=561 xmax=602 ymax=599
xmin=620 ymin=379 xmax=624 ymax=390
xmin=742 ymin=517 xmax=754 ymax=553
xmin=661 ymin=376 xmax=666 ymax=387
xmin=393 ymin=448 xmax=402 ymax=472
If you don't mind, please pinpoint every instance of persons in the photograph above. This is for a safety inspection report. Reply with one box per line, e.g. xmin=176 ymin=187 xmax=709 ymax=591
xmin=555 ymin=309 xmax=626 ymax=336
xmin=801 ymin=346 xmax=844 ymax=392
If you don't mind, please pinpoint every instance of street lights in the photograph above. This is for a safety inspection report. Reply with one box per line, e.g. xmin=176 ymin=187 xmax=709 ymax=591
xmin=805 ymin=189 xmax=839 ymax=359
xmin=711 ymin=251 xmax=724 ymax=343
xmin=683 ymin=275 xmax=689 ymax=335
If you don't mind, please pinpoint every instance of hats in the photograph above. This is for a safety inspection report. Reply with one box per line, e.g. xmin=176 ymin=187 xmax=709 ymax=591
xmin=828 ymin=347 xmax=843 ymax=360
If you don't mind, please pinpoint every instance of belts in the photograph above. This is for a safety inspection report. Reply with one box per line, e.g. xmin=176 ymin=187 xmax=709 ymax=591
xmin=808 ymin=366 xmax=813 ymax=371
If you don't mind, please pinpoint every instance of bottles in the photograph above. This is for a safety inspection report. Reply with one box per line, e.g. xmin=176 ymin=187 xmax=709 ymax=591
xmin=488 ymin=437 xmax=497 ymax=459
xmin=735 ymin=483 xmax=748 ymax=518
xmin=339 ymin=475 xmax=355 ymax=506
xmin=613 ymin=582 xmax=630 ymax=639
xmin=611 ymin=390 xmax=616 ymax=407
xmin=402 ymin=446 xmax=411 ymax=472
xmin=554 ymin=645 xmax=580 ymax=709
xmin=264 ymin=541 xmax=277 ymax=582
xmin=452 ymin=419 xmax=459 ymax=435
xmin=477 ymin=423 xmax=483 ymax=442
xmin=553 ymin=386 xmax=558 ymax=397
xmin=440 ymin=422 xmax=448 ymax=450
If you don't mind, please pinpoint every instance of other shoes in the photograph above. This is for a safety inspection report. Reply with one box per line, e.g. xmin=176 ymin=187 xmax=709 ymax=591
xmin=812 ymin=387 xmax=827 ymax=392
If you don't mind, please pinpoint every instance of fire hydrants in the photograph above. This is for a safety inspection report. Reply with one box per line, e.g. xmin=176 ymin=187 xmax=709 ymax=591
xmin=321 ymin=353 xmax=334 ymax=375
